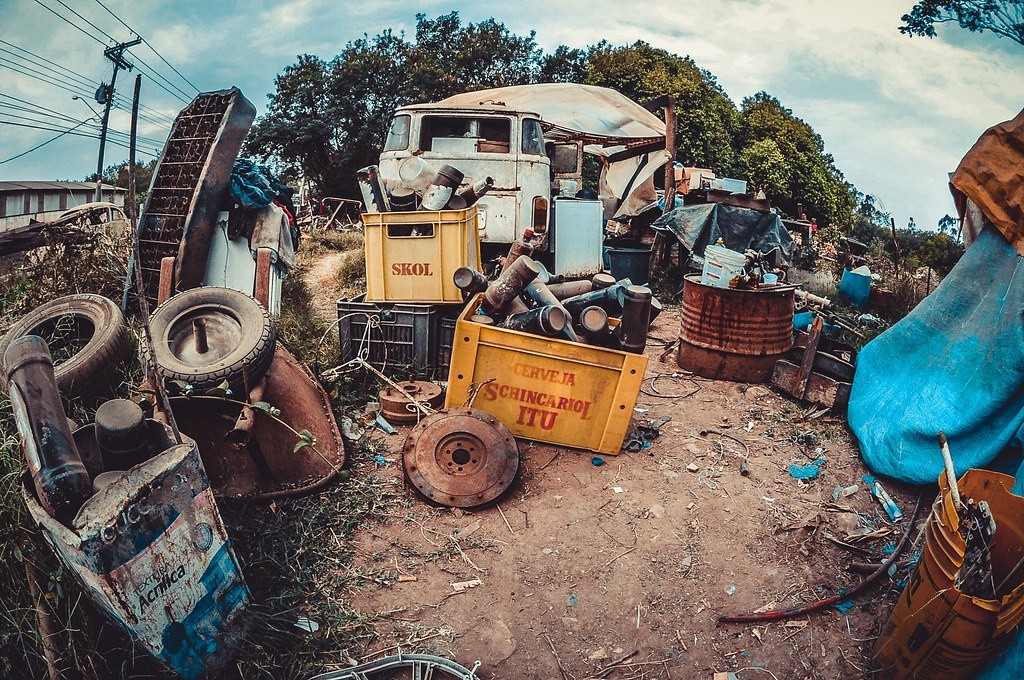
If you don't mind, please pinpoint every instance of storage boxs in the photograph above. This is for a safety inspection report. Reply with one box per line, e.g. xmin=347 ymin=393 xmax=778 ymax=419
xmin=444 ymin=291 xmax=650 ymax=457
xmin=438 ymin=310 xmax=462 ymax=383
xmin=337 ymin=293 xmax=452 ymax=385
xmin=674 ymin=165 xmax=715 ymax=196
xmin=20 ymin=417 xmax=264 ymax=680
xmin=359 ymin=201 xmax=487 ymax=304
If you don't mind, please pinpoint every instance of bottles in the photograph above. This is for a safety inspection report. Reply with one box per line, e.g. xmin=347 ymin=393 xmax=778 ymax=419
xmin=96 ymin=400 xmax=147 ymax=472
xmin=356 ymin=157 xmax=495 ymax=236
xmin=4 ymin=336 xmax=92 ymax=533
xmin=714 ymin=237 xmax=725 ymax=248
xmin=453 ymin=229 xmax=661 ymax=354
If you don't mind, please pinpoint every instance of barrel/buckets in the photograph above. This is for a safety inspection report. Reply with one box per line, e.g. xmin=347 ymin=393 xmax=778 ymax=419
xmin=763 ymin=274 xmax=778 ymax=285
xmin=559 ymin=180 xmax=577 ymax=198
xmin=839 ymin=264 xmax=871 ymax=306
xmin=702 ymin=245 xmax=745 ymax=291
